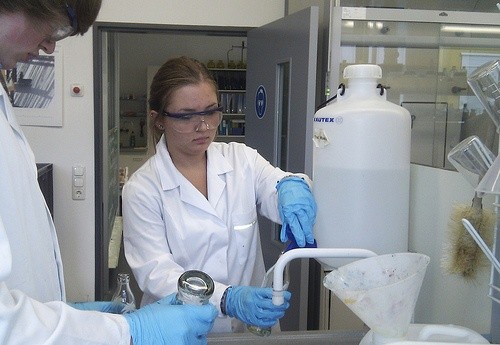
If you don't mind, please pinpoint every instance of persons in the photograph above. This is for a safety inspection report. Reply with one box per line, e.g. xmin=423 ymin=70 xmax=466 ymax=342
xmin=0 ymin=1 xmax=220 ymax=345
xmin=120 ymin=56 xmax=318 ymax=328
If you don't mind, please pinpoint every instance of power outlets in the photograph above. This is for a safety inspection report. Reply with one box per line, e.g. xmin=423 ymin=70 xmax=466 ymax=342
xmin=72 ymin=166 xmax=86 ymax=200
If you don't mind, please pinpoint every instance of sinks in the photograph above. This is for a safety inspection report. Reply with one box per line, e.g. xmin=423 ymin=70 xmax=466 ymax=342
xmin=206 ymin=330 xmax=367 ymax=345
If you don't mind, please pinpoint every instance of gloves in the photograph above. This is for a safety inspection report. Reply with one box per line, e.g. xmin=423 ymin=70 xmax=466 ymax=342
xmin=124 ymin=292 xmax=219 ymax=345
xmin=224 ymin=285 xmax=292 ymax=328
xmin=70 ymin=300 xmax=137 ymax=314
xmin=276 ymin=174 xmax=317 ymax=248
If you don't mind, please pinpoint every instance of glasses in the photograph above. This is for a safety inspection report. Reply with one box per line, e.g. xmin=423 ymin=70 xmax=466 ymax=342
xmin=162 ymin=101 xmax=224 ymax=133
xmin=49 ymin=0 xmax=78 ymax=42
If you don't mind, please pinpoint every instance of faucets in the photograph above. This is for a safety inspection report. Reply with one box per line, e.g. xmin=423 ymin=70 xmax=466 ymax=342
xmin=271 ymin=248 xmax=378 ymax=306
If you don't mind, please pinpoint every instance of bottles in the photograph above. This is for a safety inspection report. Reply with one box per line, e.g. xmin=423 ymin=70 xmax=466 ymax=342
xmin=246 ymin=246 xmax=292 ymax=336
xmin=216 ymin=60 xmax=224 ymax=68
xmin=467 ymin=62 xmax=500 ymax=127
xmin=227 ymin=45 xmax=247 ymax=69
xmin=109 ymin=272 xmax=136 ymax=315
xmin=174 ymin=270 xmax=215 ymax=304
xmin=447 ymin=136 xmax=497 ymax=196
xmin=207 ymin=59 xmax=215 ymax=68
xmin=310 ymin=61 xmax=412 ymax=269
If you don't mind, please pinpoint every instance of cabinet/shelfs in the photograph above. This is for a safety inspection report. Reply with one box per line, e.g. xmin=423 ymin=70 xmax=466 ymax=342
xmin=120 ymin=100 xmax=146 ymax=150
xmin=207 ymin=68 xmax=246 ymax=137
